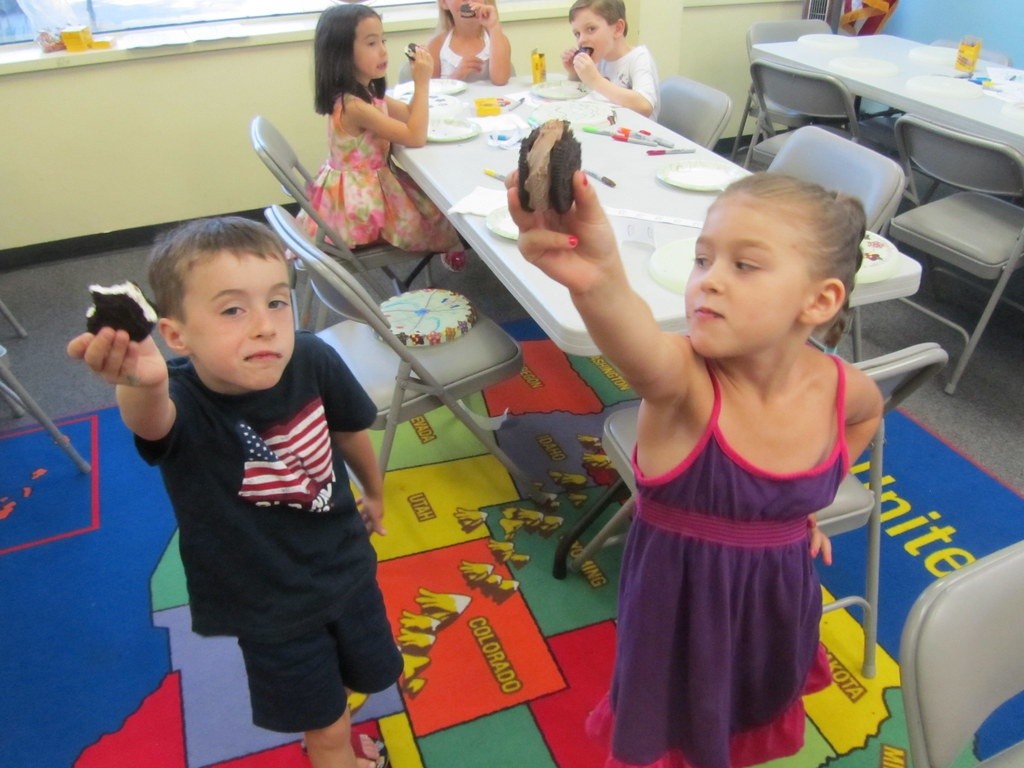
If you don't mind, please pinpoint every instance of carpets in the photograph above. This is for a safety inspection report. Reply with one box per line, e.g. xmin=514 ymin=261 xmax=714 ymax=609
xmin=0 ymin=318 xmax=1024 ymax=767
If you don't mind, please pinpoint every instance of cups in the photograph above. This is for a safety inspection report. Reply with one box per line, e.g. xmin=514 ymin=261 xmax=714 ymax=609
xmin=474 ymin=97 xmax=501 ymax=116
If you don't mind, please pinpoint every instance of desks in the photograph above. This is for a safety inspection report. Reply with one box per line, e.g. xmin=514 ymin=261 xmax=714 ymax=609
xmin=385 ymin=71 xmax=921 ymax=579
xmin=752 ymin=34 xmax=1024 ymax=157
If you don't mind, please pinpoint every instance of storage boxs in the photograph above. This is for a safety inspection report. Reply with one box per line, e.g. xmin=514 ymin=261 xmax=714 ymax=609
xmin=61 ymin=25 xmax=115 ymax=51
xmin=531 ymin=47 xmax=546 ymax=83
xmin=475 ymin=97 xmax=500 ymax=117
xmin=954 ymin=35 xmax=981 ymax=74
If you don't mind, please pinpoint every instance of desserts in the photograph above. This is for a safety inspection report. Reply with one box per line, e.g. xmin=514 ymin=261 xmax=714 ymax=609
xmin=460 ymin=4 xmax=476 ymax=18
xmin=86 ymin=280 xmax=157 ymax=343
xmin=405 ymin=43 xmax=419 ymax=60
xmin=572 ymin=48 xmax=591 ymax=56
xmin=519 ymin=120 xmax=580 ymax=213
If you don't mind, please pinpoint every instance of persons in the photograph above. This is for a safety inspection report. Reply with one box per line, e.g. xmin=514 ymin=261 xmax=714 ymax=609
xmin=561 ymin=0 xmax=661 ymax=122
xmin=67 ymin=216 xmax=404 ymax=768
xmin=398 ymin=0 xmax=517 ymax=86
xmin=504 ymin=172 xmax=883 ymax=768
xmin=285 ymin=4 xmax=465 ymax=273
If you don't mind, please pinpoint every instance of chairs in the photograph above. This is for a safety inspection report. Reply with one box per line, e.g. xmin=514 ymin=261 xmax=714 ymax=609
xmin=898 ymin=535 xmax=1024 ymax=767
xmin=566 ymin=342 xmax=949 ymax=679
xmin=263 ymin=204 xmax=551 ymax=508
xmin=250 ymin=116 xmax=438 ymax=334
xmin=657 ymin=18 xmax=1024 ymax=395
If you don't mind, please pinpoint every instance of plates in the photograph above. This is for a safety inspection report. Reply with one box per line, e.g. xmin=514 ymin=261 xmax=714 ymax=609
xmin=655 ymin=167 xmax=738 ymax=191
xmin=796 ymin=33 xmax=1023 ymax=106
xmin=426 ymin=117 xmax=481 ymax=141
xmin=405 ymin=79 xmax=467 ymax=96
xmin=485 ymin=200 xmax=518 ymax=239
xmin=531 ymin=79 xmax=592 ymax=100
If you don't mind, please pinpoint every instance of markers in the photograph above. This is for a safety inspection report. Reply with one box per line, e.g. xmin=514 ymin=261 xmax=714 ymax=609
xmin=580 ymin=125 xmax=674 ymax=148
xmin=583 ymin=169 xmax=616 ymax=188
xmin=647 ymin=149 xmax=695 ymax=154
xmin=485 ymin=169 xmax=505 ymax=180
xmin=509 ymin=97 xmax=526 ymax=112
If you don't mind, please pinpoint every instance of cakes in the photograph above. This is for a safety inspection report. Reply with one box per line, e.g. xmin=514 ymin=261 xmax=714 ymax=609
xmin=374 ymin=288 xmax=477 ymax=346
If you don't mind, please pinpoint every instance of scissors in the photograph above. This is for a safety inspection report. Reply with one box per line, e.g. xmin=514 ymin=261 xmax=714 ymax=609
xmin=972 ymin=75 xmax=1001 ymax=90
